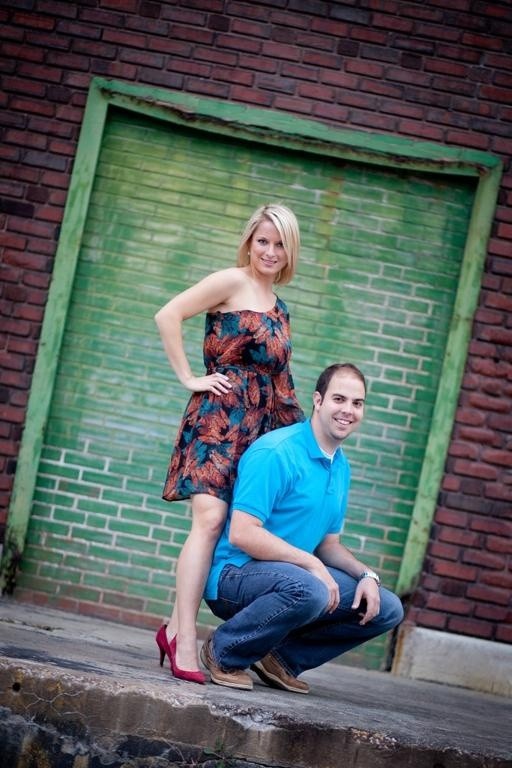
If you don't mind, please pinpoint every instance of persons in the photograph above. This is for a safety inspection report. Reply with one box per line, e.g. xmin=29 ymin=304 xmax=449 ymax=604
xmin=200 ymin=365 xmax=405 ymax=694
xmin=155 ymin=203 xmax=305 ymax=682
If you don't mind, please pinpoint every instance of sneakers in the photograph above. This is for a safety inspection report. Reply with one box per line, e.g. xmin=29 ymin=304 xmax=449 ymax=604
xmin=198 ymin=629 xmax=255 ymax=690
xmin=251 ymin=651 xmax=311 ymax=695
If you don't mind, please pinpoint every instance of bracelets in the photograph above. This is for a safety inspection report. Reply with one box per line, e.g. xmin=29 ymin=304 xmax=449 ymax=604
xmin=357 ymin=572 xmax=381 ymax=588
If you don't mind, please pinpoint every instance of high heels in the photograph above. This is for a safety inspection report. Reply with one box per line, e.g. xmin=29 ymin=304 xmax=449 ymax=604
xmin=168 ymin=634 xmax=208 ymax=685
xmin=156 ymin=622 xmax=172 ymax=668
